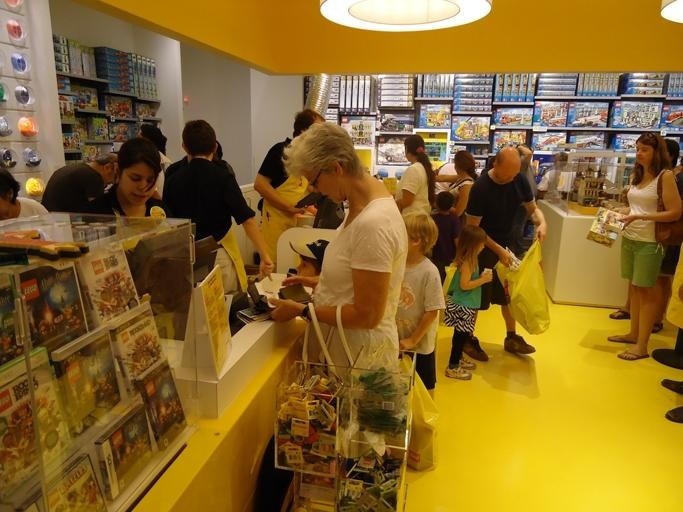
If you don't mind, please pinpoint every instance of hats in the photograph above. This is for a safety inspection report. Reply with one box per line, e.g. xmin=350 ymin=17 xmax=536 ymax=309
xmin=289 ymin=240 xmax=330 ymax=262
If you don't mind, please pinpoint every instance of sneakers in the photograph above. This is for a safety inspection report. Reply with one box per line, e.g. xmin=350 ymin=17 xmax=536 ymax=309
xmin=445 ymin=337 xmax=488 ymax=380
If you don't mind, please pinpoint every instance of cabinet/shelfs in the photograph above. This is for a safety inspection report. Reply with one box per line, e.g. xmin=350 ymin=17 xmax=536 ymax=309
xmin=56 ymin=71 xmax=162 ymax=154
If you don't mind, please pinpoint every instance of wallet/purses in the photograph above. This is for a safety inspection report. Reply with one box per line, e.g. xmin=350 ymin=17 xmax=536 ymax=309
xmin=248 ymin=284 xmax=311 ymax=308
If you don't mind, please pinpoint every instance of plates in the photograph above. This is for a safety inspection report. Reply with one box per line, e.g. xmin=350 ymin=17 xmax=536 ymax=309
xmin=261 ymin=273 xmax=287 ymax=293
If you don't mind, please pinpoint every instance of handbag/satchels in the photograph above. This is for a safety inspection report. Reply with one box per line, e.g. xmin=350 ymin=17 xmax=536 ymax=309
xmin=655 ymin=208 xmax=683 ymax=245
xmin=295 ymin=192 xmax=345 ymax=229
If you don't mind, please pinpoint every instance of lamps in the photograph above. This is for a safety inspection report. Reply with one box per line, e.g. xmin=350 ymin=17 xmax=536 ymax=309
xmin=661 ymin=0 xmax=683 ymax=24
xmin=319 ymin=0 xmax=492 ymax=32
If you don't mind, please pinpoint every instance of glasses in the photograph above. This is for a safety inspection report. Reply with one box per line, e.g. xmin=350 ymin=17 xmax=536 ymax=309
xmin=308 ymin=168 xmax=323 ymax=193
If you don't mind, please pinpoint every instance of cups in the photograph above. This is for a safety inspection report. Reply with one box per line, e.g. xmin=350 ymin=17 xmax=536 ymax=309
xmin=293 ymin=208 xmax=317 ymax=228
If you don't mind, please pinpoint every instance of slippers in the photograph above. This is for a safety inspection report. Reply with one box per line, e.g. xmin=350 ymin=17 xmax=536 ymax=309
xmin=608 ymin=310 xmax=663 ymax=360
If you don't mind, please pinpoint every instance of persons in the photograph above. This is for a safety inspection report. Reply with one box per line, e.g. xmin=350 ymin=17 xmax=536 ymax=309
xmin=536 ymin=150 xmax=607 ymax=206
xmin=1 ymin=109 xmax=547 ymax=426
xmin=602 ymin=134 xmax=682 ymax=424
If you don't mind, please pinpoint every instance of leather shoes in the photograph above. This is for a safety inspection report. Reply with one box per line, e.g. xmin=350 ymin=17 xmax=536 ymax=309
xmin=504 ymin=336 xmax=536 ymax=353
xmin=652 ymin=349 xmax=682 ymax=422
